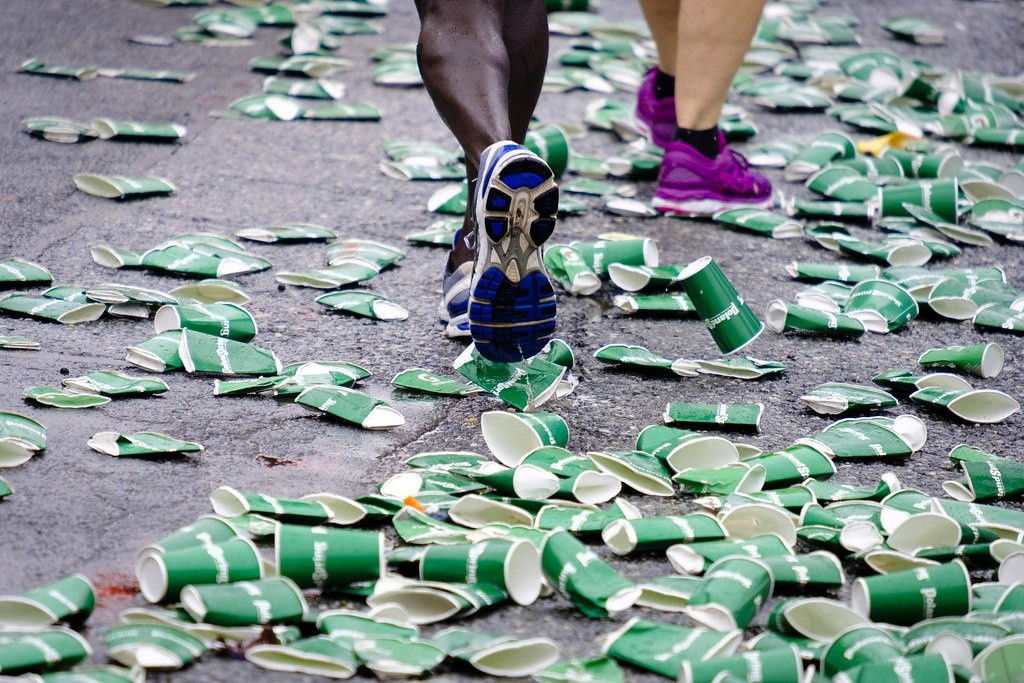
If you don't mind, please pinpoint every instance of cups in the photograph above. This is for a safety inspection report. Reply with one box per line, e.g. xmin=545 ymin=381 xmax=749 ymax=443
xmin=0 ymin=0 xmax=1024 ymax=683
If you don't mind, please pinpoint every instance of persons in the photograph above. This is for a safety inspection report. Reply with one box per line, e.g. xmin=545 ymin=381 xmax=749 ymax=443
xmin=416 ymin=1 xmax=558 ymax=363
xmin=635 ymin=2 xmax=776 ymax=214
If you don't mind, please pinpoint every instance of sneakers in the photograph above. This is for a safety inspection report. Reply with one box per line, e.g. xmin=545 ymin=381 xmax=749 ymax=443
xmin=651 ymin=126 xmax=775 ymax=216
xmin=467 ymin=140 xmax=560 ymax=364
xmin=632 ymin=67 xmax=676 ymax=140
xmin=437 ymin=227 xmax=472 ymax=338
